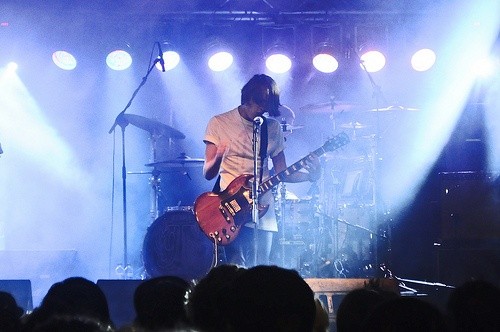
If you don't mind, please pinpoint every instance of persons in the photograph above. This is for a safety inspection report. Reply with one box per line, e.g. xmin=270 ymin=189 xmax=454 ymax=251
xmin=0 ymin=265 xmax=500 ymax=332
xmin=202 ymin=74 xmax=321 ymax=267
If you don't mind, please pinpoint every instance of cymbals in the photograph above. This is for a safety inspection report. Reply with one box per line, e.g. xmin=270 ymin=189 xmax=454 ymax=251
xmin=368 ymin=105 xmax=419 ymax=112
xmin=337 ymin=123 xmax=368 ymax=128
xmin=123 ymin=113 xmax=185 ymax=140
xmin=144 ymin=158 xmax=205 ymax=168
xmin=300 ymin=102 xmax=356 ymax=113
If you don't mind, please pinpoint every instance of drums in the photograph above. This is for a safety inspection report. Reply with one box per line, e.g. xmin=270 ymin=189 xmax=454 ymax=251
xmin=142 ymin=209 xmax=215 ymax=281
xmin=276 ymin=199 xmax=314 ymax=223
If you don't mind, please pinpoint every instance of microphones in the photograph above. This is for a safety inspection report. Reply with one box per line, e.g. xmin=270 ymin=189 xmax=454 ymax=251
xmin=157 ymin=42 xmax=165 ymax=72
xmin=253 ymin=117 xmax=264 ymax=126
xmin=345 ymin=34 xmax=350 ymax=59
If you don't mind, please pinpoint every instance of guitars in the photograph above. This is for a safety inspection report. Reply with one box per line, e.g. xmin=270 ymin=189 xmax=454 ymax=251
xmin=194 ymin=130 xmax=351 ymax=246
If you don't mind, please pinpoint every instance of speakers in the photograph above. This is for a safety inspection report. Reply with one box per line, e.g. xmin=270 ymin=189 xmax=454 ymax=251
xmin=0 ymin=248 xmax=85 ymax=316
xmin=95 ymin=278 xmax=145 ymax=332
xmin=437 ymin=170 xmax=500 ymax=249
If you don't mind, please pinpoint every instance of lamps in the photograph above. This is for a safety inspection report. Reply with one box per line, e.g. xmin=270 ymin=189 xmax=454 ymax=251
xmin=51 ymin=15 xmax=436 ymax=74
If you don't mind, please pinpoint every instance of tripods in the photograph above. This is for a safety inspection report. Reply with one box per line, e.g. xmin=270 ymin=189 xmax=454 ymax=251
xmin=312 ymin=46 xmax=406 ymax=290
